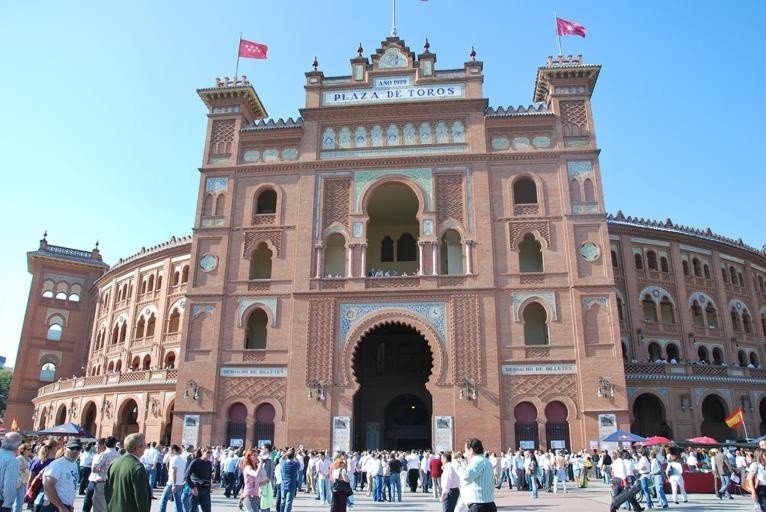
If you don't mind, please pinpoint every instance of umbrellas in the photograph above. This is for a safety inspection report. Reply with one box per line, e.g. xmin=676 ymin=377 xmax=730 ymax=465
xmin=33 ymin=421 xmax=94 ymax=443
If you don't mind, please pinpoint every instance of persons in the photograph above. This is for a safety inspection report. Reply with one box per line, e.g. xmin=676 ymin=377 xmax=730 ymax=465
xmin=623 ymin=355 xmax=765 ymax=369
xmin=324 ymin=264 xmax=421 ymax=277
xmin=555 ymin=17 xmax=586 ymax=39
xmin=55 ymin=361 xmax=175 ymax=383
xmin=238 ymin=38 xmax=269 ymax=60
xmin=1 ymin=432 xmax=766 ymax=511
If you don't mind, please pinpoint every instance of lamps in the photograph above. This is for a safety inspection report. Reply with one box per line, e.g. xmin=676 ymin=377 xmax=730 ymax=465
xmin=307 ymin=379 xmax=326 ymax=402
xmin=183 ymin=379 xmax=200 ymax=401
xmin=459 ymin=378 xmax=477 ymax=402
xmin=597 ymin=376 xmax=615 ymax=402
xmin=636 ymin=326 xmax=741 ymax=349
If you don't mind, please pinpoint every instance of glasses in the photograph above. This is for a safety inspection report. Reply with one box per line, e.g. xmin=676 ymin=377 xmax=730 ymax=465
xmin=207 ymin=451 xmax=212 ymax=454
xmin=24 ymin=447 xmax=31 ymax=451
xmin=69 ymin=448 xmax=80 ymax=451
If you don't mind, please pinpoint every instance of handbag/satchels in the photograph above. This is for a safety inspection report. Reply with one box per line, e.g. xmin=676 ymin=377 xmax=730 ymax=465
xmin=553 ymin=457 xmax=557 ymax=476
xmin=333 ymin=469 xmax=353 ymax=496
xmin=743 ymin=462 xmax=761 ymax=493
xmin=26 ymin=476 xmax=42 ymax=503
xmin=668 ymin=463 xmax=674 ymax=476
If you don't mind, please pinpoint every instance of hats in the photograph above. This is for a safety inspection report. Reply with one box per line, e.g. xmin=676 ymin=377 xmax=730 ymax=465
xmin=66 ymin=438 xmax=81 ymax=449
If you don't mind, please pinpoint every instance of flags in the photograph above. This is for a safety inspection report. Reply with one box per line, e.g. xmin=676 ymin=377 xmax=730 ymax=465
xmin=10 ymin=416 xmax=20 ymax=432
xmin=723 ymin=406 xmax=744 ymax=432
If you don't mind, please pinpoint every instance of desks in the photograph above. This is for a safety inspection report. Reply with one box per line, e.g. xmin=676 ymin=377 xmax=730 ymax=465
xmin=662 ymin=468 xmax=753 ymax=494
